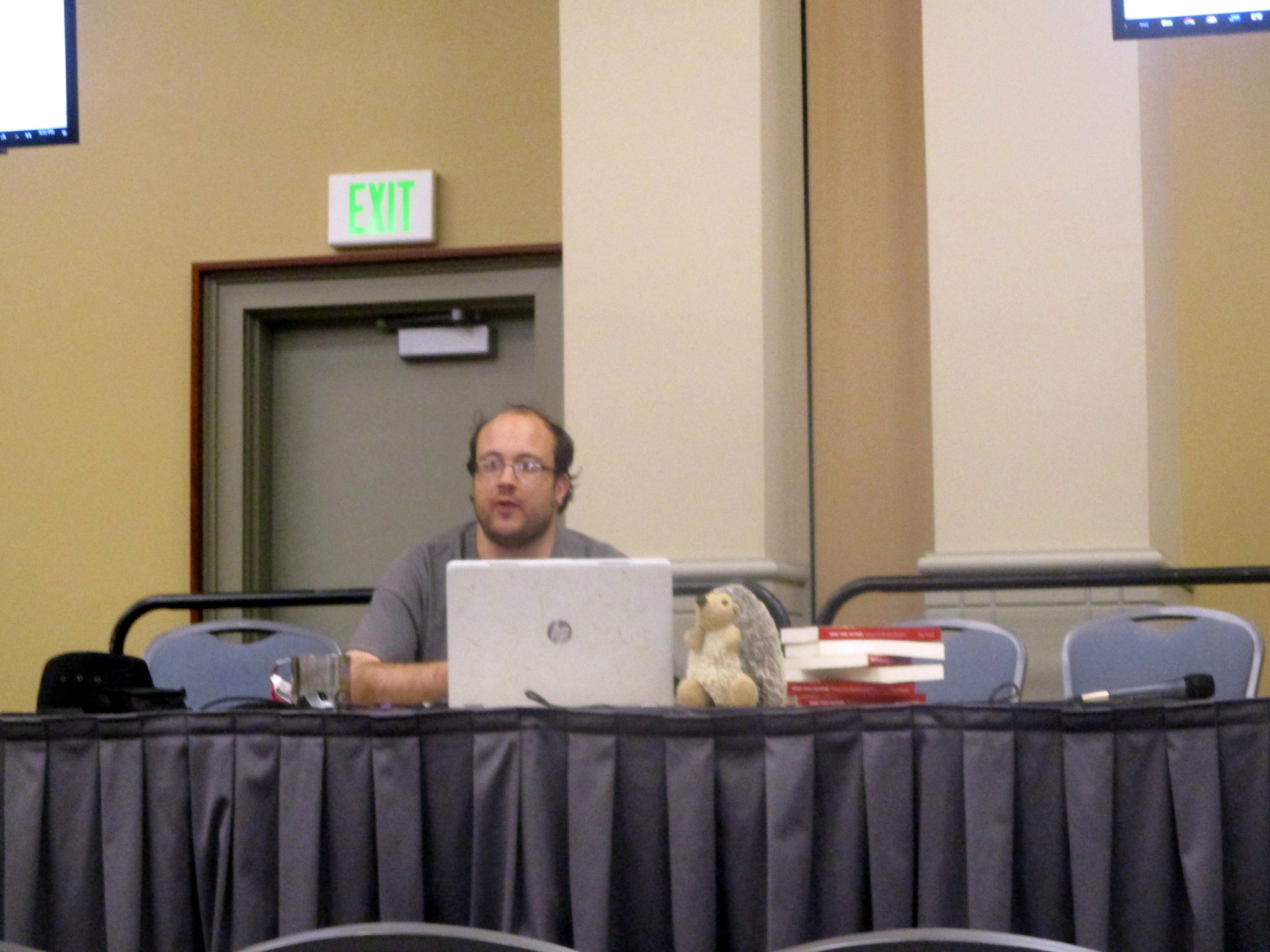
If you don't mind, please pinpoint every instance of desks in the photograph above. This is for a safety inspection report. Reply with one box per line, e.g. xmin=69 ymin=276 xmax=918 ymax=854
xmin=0 ymin=706 xmax=1270 ymax=952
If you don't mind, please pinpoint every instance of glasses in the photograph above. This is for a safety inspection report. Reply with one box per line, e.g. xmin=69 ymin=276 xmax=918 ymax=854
xmin=475 ymin=456 xmax=558 ymax=476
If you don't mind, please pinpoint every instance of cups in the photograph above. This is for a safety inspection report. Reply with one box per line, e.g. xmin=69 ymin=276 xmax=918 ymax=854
xmin=290 ymin=655 xmax=351 ymax=713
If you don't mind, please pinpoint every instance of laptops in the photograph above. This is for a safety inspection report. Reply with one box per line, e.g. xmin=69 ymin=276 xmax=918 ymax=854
xmin=443 ymin=557 xmax=675 ymax=709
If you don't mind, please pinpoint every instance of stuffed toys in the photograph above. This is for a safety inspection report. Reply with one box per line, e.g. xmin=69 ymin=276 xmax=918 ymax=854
xmin=675 ymin=584 xmax=788 ymax=709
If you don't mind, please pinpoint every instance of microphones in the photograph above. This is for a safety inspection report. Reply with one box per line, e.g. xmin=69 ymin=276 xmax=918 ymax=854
xmin=1077 ymin=673 xmax=1217 ymax=702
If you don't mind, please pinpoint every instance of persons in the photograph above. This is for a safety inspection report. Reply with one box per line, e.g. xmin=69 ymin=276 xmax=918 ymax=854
xmin=343 ymin=404 xmax=630 ymax=708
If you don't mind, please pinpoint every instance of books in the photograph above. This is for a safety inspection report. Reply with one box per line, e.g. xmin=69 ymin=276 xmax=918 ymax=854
xmin=773 ymin=624 xmax=946 ymax=710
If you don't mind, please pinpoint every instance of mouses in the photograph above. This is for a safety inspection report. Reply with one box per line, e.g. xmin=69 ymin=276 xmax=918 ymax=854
xmin=300 ymin=689 xmax=337 ymax=711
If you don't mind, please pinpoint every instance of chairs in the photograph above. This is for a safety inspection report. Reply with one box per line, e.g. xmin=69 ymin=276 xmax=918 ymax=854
xmin=904 ymin=618 xmax=1028 ymax=704
xmin=145 ymin=619 xmax=344 ymax=709
xmin=1062 ymin=608 xmax=1265 ymax=701
